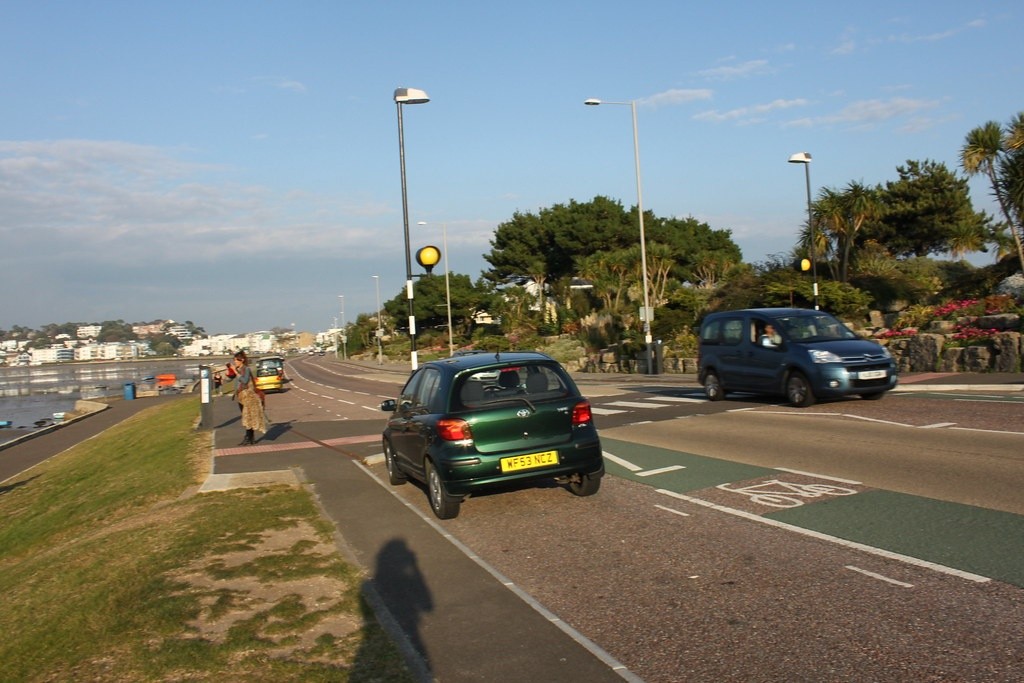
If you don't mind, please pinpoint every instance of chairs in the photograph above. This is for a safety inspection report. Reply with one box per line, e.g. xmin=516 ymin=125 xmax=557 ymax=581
xmin=494 ymin=370 xmax=525 ymax=397
xmin=526 ymin=374 xmax=548 ymax=394
xmin=461 ymin=381 xmax=484 ymax=403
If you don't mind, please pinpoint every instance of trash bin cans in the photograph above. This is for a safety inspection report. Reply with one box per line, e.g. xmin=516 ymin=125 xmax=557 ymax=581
xmin=123 ymin=382 xmax=135 ymax=400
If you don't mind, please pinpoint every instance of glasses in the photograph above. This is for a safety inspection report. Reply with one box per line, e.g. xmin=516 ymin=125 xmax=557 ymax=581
xmin=767 ymin=327 xmax=775 ymax=329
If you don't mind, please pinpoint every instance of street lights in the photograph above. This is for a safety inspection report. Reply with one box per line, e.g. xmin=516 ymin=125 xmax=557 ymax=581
xmin=394 ymin=85 xmax=432 ymax=374
xmin=333 ymin=317 xmax=338 ymax=358
xmin=585 ymin=98 xmax=655 ymax=376
xmin=416 ymin=221 xmax=454 ymax=358
xmin=338 ymin=295 xmax=347 ymax=361
xmin=789 ymin=152 xmax=819 ymax=310
xmin=371 ymin=275 xmax=383 ymax=362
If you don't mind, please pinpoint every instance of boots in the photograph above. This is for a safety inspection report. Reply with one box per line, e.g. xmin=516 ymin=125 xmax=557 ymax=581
xmin=238 ymin=436 xmax=251 ymax=446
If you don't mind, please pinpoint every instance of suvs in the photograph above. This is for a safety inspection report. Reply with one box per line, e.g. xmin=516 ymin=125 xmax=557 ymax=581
xmin=255 ymin=355 xmax=284 ymax=376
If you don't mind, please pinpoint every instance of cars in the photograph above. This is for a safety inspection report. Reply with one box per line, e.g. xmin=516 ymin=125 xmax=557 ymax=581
xmin=308 ymin=349 xmax=326 ymax=357
xmin=698 ymin=308 xmax=896 ymax=406
xmin=252 ymin=367 xmax=282 ymax=392
xmin=378 ymin=353 xmax=606 ymax=519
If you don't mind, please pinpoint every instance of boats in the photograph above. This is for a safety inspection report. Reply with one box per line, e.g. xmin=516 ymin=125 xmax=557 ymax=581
xmin=155 ymin=373 xmax=176 ymax=381
